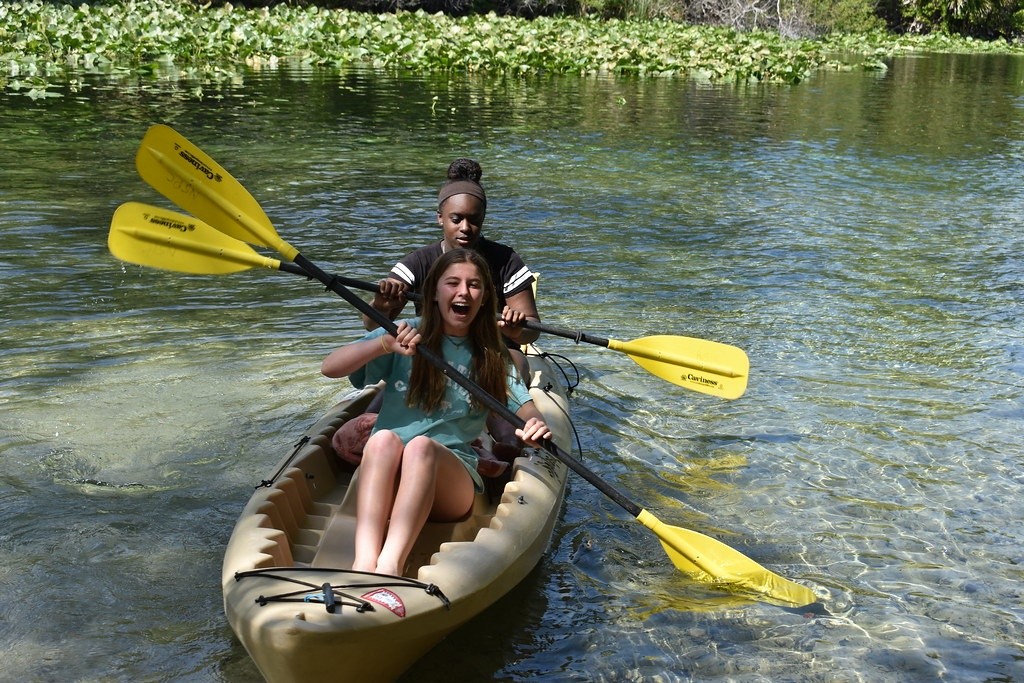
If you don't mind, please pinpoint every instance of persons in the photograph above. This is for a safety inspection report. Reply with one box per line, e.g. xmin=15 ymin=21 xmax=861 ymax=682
xmin=358 ymin=159 xmax=540 ymax=414
xmin=321 ymin=245 xmax=553 ymax=575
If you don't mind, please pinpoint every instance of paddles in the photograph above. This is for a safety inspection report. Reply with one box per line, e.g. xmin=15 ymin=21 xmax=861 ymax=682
xmin=134 ymin=122 xmax=820 ymax=610
xmin=103 ymin=200 xmax=751 ymax=402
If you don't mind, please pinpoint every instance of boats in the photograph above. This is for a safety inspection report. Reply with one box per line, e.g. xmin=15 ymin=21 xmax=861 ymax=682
xmin=220 ymin=334 xmax=572 ymax=683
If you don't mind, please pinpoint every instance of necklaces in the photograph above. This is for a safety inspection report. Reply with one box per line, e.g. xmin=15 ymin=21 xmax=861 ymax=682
xmin=446 ymin=335 xmax=466 ymax=349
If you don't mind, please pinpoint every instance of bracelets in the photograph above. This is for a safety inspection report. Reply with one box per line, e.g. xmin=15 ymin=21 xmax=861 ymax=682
xmin=381 ymin=335 xmax=391 ymax=354
